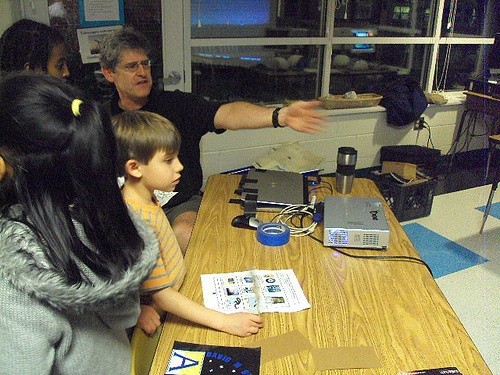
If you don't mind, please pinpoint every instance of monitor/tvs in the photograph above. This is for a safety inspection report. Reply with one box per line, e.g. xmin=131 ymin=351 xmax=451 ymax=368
xmin=350 ymin=28 xmax=376 ymax=53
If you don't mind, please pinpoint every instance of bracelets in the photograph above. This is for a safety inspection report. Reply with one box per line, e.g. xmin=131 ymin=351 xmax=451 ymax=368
xmin=272 ymin=107 xmax=285 ymax=130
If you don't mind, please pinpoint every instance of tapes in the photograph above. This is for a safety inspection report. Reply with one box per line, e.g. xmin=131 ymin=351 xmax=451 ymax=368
xmin=258 ymin=222 xmax=290 ymax=247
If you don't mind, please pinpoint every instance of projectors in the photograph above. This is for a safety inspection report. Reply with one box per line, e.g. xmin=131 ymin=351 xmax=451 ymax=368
xmin=323 ymin=196 xmax=390 ymax=249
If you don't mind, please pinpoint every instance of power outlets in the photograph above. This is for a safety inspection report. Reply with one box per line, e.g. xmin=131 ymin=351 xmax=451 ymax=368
xmin=414 ymin=116 xmax=424 ymax=129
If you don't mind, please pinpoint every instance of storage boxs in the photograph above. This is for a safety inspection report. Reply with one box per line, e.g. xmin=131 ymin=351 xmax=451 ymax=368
xmin=357 ymin=167 xmax=438 ymax=222
xmin=382 ymin=162 xmax=416 ymax=180
xmin=381 ymin=145 xmax=442 ymax=169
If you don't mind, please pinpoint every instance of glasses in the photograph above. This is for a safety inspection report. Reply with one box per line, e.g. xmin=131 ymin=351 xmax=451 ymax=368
xmin=114 ymin=59 xmax=152 ymax=72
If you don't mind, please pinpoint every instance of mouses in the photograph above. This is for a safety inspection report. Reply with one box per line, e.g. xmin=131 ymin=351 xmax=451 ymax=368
xmin=232 ymin=215 xmax=262 ymax=230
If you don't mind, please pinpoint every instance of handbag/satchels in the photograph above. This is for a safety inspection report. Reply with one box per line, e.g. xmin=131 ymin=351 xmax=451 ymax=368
xmin=378 ymin=76 xmax=429 ymax=126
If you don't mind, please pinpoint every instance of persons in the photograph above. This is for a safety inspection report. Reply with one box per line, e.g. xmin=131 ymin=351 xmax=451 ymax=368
xmin=0 ymin=70 xmax=159 ymax=375
xmin=0 ymin=19 xmax=71 ymax=86
xmin=99 ymin=27 xmax=327 ymax=254
xmin=137 ymin=304 xmax=161 ymax=336
xmin=109 ymin=109 xmax=263 ymax=338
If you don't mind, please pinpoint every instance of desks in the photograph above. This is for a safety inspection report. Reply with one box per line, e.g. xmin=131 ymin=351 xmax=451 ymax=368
xmin=193 ymin=58 xmax=410 ymax=99
xmin=145 ymin=175 xmax=493 ymax=375
xmin=449 ymin=91 xmax=500 ymax=166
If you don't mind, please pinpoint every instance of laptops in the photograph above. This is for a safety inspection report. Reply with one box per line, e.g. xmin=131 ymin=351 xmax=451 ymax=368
xmin=239 ymin=168 xmax=303 ymax=207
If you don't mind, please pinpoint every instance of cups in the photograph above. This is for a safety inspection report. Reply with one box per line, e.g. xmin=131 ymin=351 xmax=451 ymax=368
xmin=335 ymin=147 xmax=357 ymax=194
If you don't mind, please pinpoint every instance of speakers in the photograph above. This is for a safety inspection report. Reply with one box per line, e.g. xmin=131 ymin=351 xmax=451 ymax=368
xmin=379 ymin=145 xmax=439 ymax=223
